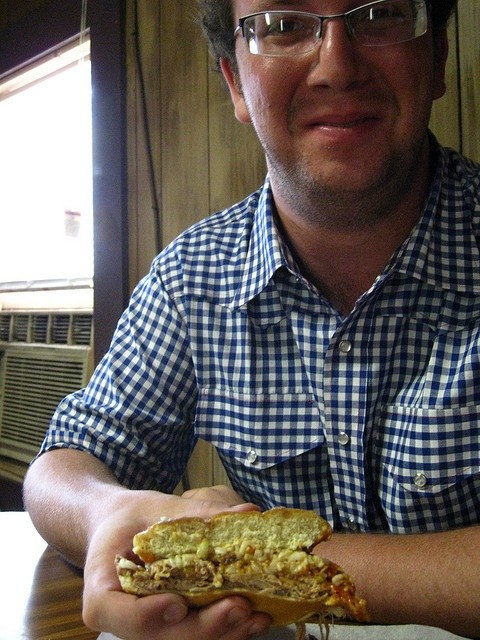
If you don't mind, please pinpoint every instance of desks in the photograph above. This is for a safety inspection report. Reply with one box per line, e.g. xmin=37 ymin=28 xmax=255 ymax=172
xmin=0 ymin=509 xmax=477 ymax=639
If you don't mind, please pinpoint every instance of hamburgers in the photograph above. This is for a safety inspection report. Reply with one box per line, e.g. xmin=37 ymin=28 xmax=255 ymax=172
xmin=114 ymin=507 xmax=370 ymax=636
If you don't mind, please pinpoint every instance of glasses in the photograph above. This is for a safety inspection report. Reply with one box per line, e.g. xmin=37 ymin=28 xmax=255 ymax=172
xmin=233 ymin=1 xmax=428 ymax=57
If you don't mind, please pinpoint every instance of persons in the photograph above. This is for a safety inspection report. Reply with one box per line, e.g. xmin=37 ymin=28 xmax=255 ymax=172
xmin=21 ymin=0 xmax=480 ymax=640
